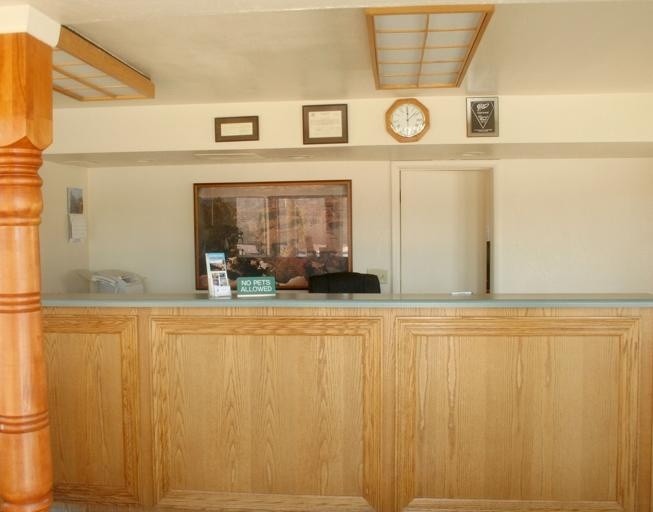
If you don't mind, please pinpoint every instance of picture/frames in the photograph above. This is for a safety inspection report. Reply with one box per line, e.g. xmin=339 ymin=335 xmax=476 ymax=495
xmin=303 ymin=103 xmax=347 ymax=144
xmin=214 ymin=116 xmax=258 ymax=142
xmin=464 ymin=96 xmax=500 ymax=138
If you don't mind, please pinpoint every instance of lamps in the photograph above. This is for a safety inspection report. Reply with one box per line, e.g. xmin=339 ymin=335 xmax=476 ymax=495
xmin=366 ymin=5 xmax=494 ymax=90
xmin=43 ymin=24 xmax=155 ymax=103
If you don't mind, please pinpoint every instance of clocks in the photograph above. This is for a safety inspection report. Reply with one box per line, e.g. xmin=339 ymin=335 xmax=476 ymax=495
xmin=385 ymin=98 xmax=432 ymax=144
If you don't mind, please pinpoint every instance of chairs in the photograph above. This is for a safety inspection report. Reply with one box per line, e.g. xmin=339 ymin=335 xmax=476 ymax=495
xmin=308 ymin=271 xmax=382 ymax=294
